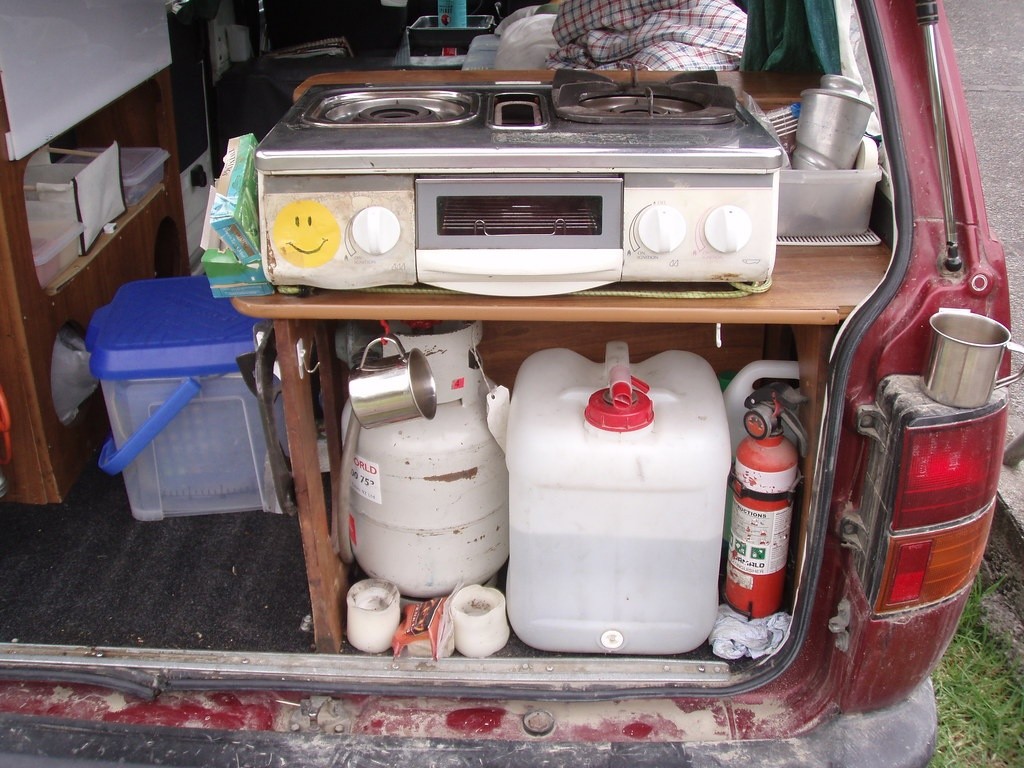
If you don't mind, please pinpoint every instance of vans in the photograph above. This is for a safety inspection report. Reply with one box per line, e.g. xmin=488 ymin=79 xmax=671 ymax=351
xmin=0 ymin=1 xmax=1024 ymax=768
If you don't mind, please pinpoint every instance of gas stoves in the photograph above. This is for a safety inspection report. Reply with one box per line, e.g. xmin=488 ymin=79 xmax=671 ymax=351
xmin=255 ymin=81 xmax=785 ymax=297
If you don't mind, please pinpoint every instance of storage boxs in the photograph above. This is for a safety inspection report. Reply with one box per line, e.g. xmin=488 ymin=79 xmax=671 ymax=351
xmin=21 ymin=145 xmax=171 ymax=290
xmin=85 ymin=277 xmax=290 ymax=522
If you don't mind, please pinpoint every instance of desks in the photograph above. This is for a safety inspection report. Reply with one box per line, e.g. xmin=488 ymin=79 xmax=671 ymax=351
xmin=231 ymin=70 xmax=895 ymax=654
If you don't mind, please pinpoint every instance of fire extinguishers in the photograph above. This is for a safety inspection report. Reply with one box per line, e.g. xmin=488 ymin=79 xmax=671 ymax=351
xmin=724 ymin=381 xmax=808 ymax=620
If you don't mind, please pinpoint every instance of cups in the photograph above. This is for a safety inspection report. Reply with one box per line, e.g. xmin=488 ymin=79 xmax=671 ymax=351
xmin=348 ymin=334 xmax=437 ymax=429
xmin=920 ymin=311 xmax=1024 ymax=408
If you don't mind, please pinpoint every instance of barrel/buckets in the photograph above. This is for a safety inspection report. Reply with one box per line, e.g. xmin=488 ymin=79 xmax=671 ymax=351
xmin=505 ymin=341 xmax=732 ymax=655
xmin=723 ymin=360 xmax=800 ymax=542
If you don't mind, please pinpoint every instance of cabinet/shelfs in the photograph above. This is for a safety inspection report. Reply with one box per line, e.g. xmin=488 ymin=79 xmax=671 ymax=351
xmin=0 ymin=1 xmax=214 ymax=506
